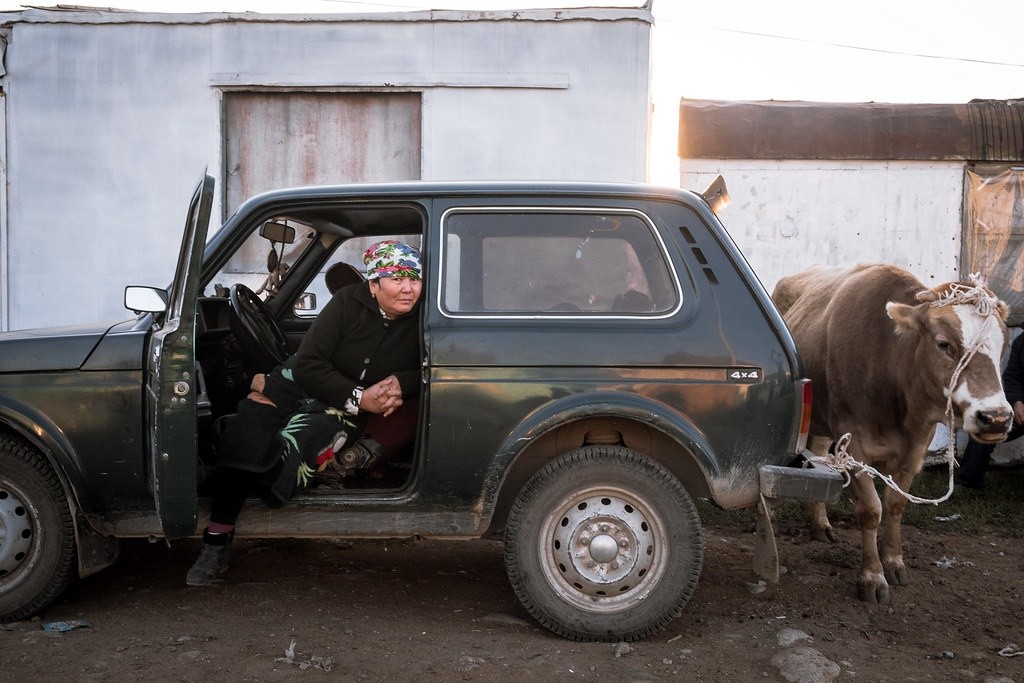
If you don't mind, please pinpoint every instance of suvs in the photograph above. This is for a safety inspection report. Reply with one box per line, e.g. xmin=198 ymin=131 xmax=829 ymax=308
xmin=0 ymin=161 xmax=844 ymax=645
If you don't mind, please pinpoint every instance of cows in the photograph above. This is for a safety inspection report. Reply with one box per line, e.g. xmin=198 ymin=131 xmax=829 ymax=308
xmin=770 ymin=262 xmax=1017 ymax=609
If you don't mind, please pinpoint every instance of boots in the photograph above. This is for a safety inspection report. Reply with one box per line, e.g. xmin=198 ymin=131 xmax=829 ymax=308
xmin=186 ymin=527 xmax=235 ymax=586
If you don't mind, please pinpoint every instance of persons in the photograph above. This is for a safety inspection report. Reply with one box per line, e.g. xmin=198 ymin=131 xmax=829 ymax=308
xmin=957 ymin=322 xmax=1024 ymax=493
xmin=187 ymin=240 xmax=425 ymax=587
